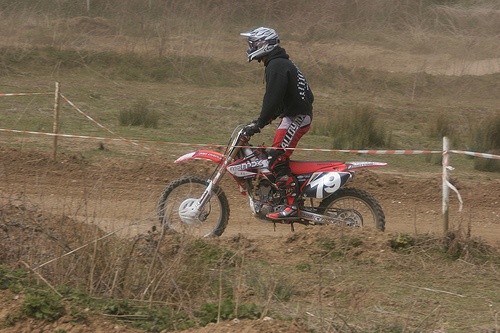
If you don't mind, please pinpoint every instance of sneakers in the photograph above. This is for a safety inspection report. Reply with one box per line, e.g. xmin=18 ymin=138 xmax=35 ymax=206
xmin=267 ymin=202 xmax=300 ymax=220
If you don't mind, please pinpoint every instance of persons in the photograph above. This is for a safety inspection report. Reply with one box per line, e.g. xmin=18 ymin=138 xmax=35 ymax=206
xmin=240 ymin=27 xmax=314 ymax=220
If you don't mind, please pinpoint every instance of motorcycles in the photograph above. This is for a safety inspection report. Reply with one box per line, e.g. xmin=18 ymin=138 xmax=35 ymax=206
xmin=156 ymin=125 xmax=389 ymax=239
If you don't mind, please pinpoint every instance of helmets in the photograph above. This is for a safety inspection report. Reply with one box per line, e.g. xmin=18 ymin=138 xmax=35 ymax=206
xmin=240 ymin=26 xmax=280 ymax=63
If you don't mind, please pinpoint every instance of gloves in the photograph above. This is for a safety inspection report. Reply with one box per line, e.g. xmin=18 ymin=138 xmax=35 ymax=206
xmin=246 ymin=120 xmax=257 ymax=128
xmin=243 ymin=125 xmax=261 ymax=136
